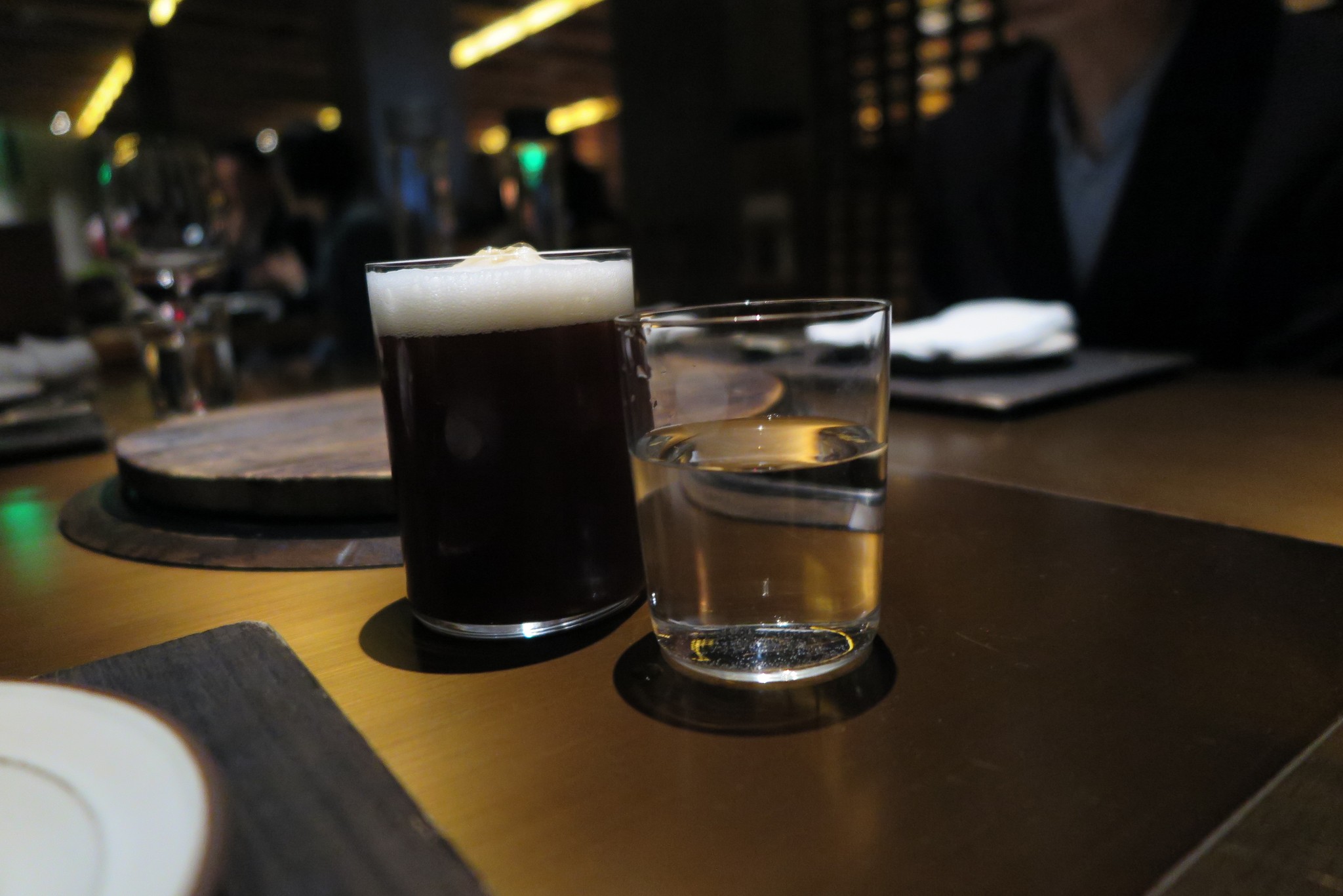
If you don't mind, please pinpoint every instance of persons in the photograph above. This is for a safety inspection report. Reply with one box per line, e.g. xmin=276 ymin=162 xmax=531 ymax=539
xmin=246 ymin=127 xmax=391 ymax=392
xmin=923 ymin=0 xmax=1343 ymax=375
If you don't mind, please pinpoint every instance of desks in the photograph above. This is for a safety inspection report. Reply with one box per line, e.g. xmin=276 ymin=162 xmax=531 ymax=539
xmin=0 ymin=333 xmax=1343 ymax=895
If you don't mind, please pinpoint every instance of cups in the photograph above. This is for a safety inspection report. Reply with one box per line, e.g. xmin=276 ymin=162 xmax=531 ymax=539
xmin=365 ymin=246 xmax=642 ymax=641
xmin=615 ymin=297 xmax=892 ymax=691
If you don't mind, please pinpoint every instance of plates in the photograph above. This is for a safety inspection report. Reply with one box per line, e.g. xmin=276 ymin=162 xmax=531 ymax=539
xmin=0 ymin=677 xmax=219 ymax=896
xmin=808 ymin=324 xmax=1073 ymax=360
